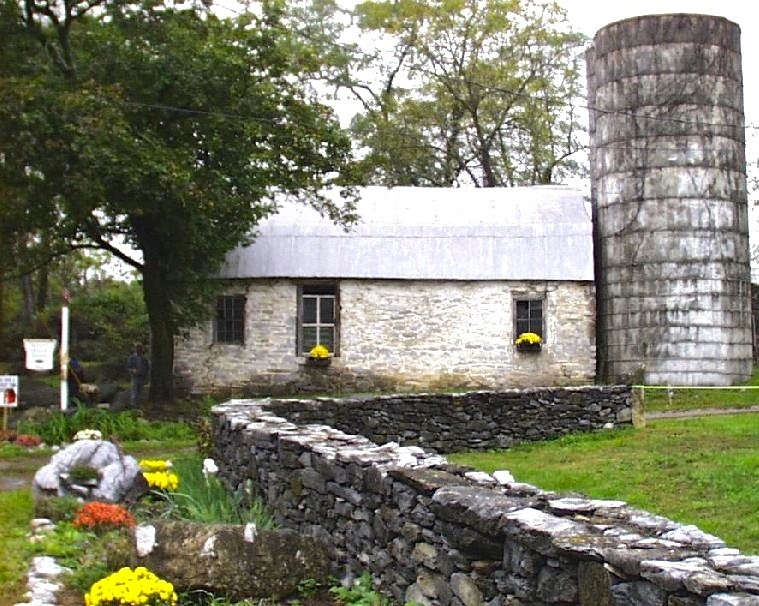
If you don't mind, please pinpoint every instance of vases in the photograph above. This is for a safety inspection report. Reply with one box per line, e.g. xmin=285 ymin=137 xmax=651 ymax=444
xmin=518 ymin=342 xmax=540 ymax=351
xmin=310 ymin=357 xmax=329 ymax=366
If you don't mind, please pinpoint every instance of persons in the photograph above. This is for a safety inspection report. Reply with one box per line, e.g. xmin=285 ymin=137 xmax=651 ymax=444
xmin=4 ymin=389 xmax=16 ymax=404
xmin=126 ymin=344 xmax=150 ymax=408
xmin=69 ymin=355 xmax=81 ymax=397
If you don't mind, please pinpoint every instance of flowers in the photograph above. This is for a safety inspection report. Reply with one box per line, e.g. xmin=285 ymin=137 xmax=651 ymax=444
xmin=310 ymin=345 xmax=330 ymax=357
xmin=515 ymin=333 xmax=542 ymax=344
